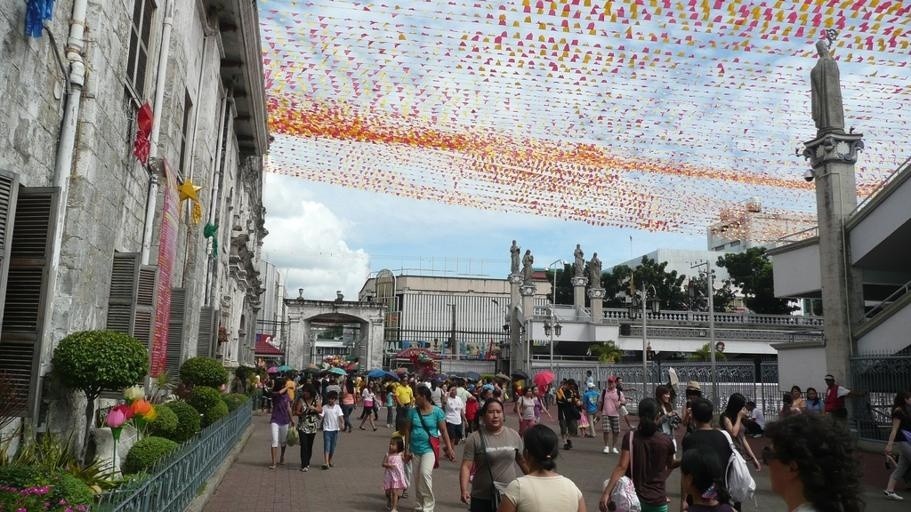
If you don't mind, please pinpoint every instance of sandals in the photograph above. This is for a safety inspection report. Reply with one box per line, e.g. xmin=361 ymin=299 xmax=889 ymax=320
xmin=321 ymin=461 xmax=336 ymax=468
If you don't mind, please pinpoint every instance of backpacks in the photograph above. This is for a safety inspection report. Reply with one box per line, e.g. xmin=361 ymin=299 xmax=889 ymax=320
xmin=717 ymin=430 xmax=756 ymax=504
xmin=555 ymin=385 xmax=568 ymax=407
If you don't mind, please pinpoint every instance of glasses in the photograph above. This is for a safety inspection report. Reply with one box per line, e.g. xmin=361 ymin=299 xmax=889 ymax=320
xmin=761 ymin=445 xmax=792 ymax=467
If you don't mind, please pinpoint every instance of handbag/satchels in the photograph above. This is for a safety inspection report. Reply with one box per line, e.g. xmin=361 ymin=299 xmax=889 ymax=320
xmin=513 ymin=395 xmax=524 ymax=416
xmin=901 ymin=429 xmax=911 ymax=445
xmin=504 ymin=392 xmax=510 ymax=401
xmin=372 ymin=396 xmax=378 ymax=413
xmin=492 ymin=479 xmax=510 ymax=512
xmin=601 ymin=473 xmax=643 ymax=511
xmin=287 ymin=425 xmax=299 ymax=447
xmin=429 ymin=434 xmax=440 ymax=469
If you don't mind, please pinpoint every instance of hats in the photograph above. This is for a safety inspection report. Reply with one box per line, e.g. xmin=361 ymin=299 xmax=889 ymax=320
xmin=684 ymin=380 xmax=702 ymax=393
xmin=607 ymin=375 xmax=617 ymax=384
xmin=587 ymin=383 xmax=596 ymax=388
xmin=824 ymin=375 xmax=835 ymax=380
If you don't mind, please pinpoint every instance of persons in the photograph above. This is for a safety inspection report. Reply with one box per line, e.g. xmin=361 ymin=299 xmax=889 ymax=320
xmin=589 ymin=252 xmax=603 ymax=287
xmin=810 ymin=39 xmax=848 ymax=138
xmin=255 ymin=361 xmax=907 ymax=511
xmin=523 ymin=249 xmax=535 ymax=285
xmin=574 ymin=243 xmax=585 ymax=277
xmin=510 ymin=239 xmax=521 ymax=274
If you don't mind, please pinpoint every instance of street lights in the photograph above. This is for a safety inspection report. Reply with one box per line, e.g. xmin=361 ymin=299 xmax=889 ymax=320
xmin=630 ymin=280 xmax=662 ymax=403
xmin=446 ymin=302 xmax=457 ymax=358
xmin=544 ymin=257 xmax=570 ymax=372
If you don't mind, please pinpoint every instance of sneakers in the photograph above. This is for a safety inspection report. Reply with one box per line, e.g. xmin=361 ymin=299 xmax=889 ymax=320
xmin=359 ymin=425 xmax=377 ymax=432
xmin=386 ymin=422 xmax=394 ymax=429
xmin=611 ymin=447 xmax=620 ymax=454
xmin=402 ymin=492 xmax=408 ymax=498
xmin=628 ymin=424 xmax=638 ymax=430
xmin=581 ymin=431 xmax=597 ymax=438
xmin=746 ymin=432 xmax=763 ymax=439
xmin=269 ymin=464 xmax=277 ymax=469
xmin=384 ymin=502 xmax=399 ymax=512
xmin=563 ymin=439 xmax=572 ymax=450
xmin=602 ymin=447 xmax=610 ymax=454
xmin=299 ymin=464 xmax=309 ymax=472
xmin=280 ymin=455 xmax=285 ymax=465
xmin=343 ymin=423 xmax=354 ymax=434
xmin=881 ymin=488 xmax=905 ymax=502
